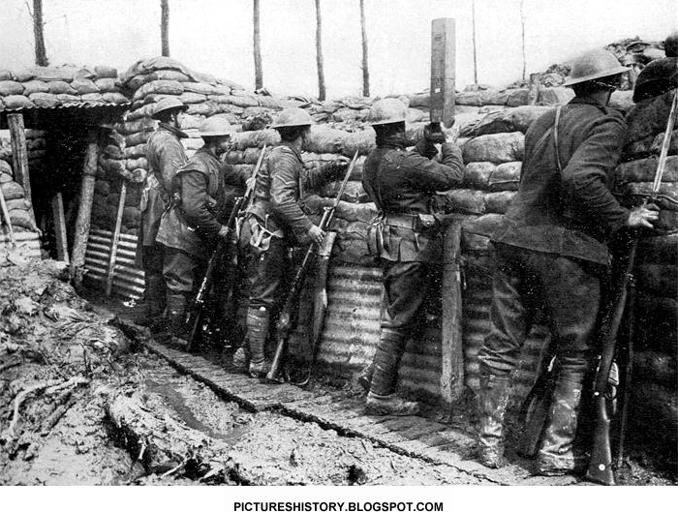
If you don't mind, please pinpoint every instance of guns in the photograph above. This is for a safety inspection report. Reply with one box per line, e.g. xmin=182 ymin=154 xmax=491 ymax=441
xmin=265 ymin=149 xmax=359 ymax=385
xmin=513 ymin=354 xmax=555 ymax=459
xmin=583 ymin=94 xmax=676 ymax=485
xmin=184 ymin=142 xmax=267 ymax=352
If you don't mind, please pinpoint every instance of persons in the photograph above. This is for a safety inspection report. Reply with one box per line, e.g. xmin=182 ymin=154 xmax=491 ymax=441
xmin=130 ymin=97 xmax=190 ymax=325
xmin=158 ymin=114 xmax=251 ymax=341
xmin=232 ymin=105 xmax=349 ymax=381
xmin=357 ymin=96 xmax=466 ymax=419
xmin=477 ymin=40 xmax=661 ymax=476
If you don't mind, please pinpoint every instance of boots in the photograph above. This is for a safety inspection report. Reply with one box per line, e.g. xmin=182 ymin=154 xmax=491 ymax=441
xmin=478 ymin=373 xmax=510 ymax=469
xmin=536 ymin=380 xmax=590 ymax=475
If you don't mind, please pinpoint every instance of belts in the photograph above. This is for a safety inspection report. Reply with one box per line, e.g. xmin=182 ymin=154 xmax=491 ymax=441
xmin=378 ymin=210 xmax=434 ymax=232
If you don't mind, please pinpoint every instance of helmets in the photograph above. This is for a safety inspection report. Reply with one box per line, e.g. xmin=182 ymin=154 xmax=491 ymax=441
xmin=151 ymin=97 xmax=188 ymax=119
xmin=367 ymin=98 xmax=407 ymax=126
xmin=563 ymin=48 xmax=631 ymax=88
xmin=271 ymin=107 xmax=313 ymax=127
xmin=198 ymin=117 xmax=234 ymax=137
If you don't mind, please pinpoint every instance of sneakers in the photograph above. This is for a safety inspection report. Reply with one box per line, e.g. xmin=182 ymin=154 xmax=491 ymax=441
xmin=367 ymin=393 xmax=420 ymax=415
xmin=248 ymin=361 xmax=281 ymax=379
